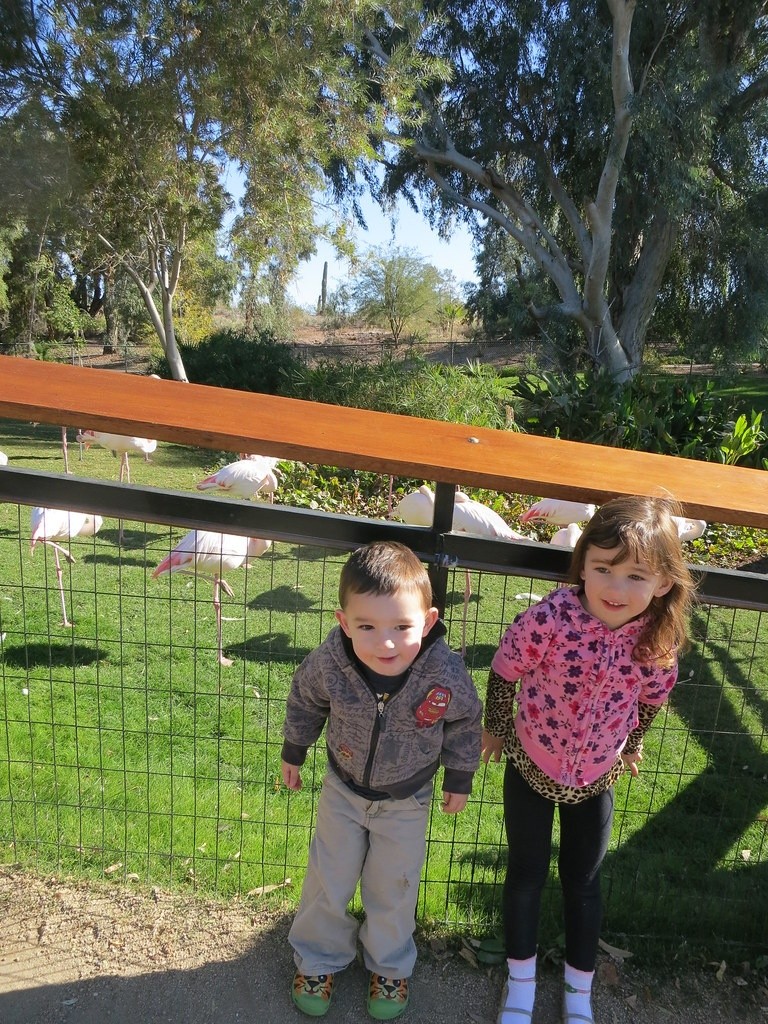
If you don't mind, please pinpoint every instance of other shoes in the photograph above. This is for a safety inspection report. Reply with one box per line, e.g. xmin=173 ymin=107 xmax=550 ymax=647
xmin=367 ymin=971 xmax=409 ymax=1020
xmin=562 ymin=982 xmax=595 ymax=1024
xmin=496 ymin=975 xmax=536 ymax=1024
xmin=291 ymin=968 xmax=334 ymax=1015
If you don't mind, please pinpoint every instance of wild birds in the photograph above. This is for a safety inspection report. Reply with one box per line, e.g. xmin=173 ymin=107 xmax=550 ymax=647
xmin=153 ymin=529 xmax=275 ymax=667
xmin=31 ymin=373 xmax=162 ymax=482
xmin=196 ymin=453 xmax=287 ymax=505
xmin=384 ymin=473 xmax=537 ymax=657
xmin=514 ymin=496 xmax=708 ymax=618
xmin=28 ymin=504 xmax=103 ymax=627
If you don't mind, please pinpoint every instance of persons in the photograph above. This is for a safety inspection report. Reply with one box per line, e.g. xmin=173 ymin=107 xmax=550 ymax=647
xmin=281 ymin=543 xmax=484 ymax=1020
xmin=480 ymin=495 xmax=688 ymax=1024
